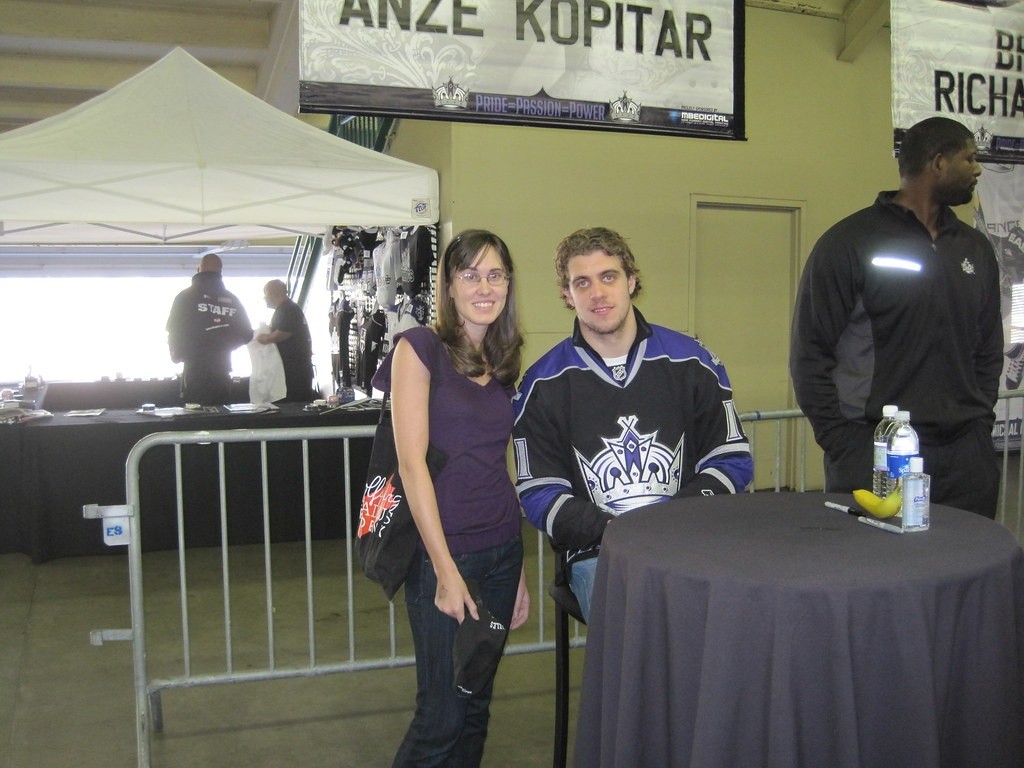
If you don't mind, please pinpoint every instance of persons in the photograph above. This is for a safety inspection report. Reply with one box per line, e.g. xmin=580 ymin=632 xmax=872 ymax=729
xmin=510 ymin=227 xmax=753 ymax=627
xmin=370 ymin=228 xmax=531 ymax=767
xmin=165 ymin=253 xmax=253 ymax=404
xmin=788 ymin=116 xmax=1005 ymax=519
xmin=256 ymin=279 xmax=314 ymax=402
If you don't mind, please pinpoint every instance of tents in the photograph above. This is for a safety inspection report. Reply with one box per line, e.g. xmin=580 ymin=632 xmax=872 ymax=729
xmin=0 ymin=45 xmax=440 ymax=248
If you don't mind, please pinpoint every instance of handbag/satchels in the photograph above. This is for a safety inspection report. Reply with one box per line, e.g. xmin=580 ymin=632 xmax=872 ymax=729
xmin=352 ymin=391 xmax=432 ymax=599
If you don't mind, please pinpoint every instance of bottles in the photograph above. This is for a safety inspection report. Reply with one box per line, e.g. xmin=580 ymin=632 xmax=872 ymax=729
xmin=886 ymin=410 xmax=920 ymax=517
xmin=873 ymin=403 xmax=901 ymax=498
xmin=902 ymin=457 xmax=931 ymax=532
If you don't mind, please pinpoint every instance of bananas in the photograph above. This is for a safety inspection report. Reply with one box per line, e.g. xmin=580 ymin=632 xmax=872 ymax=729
xmin=852 ymin=476 xmax=902 ymax=519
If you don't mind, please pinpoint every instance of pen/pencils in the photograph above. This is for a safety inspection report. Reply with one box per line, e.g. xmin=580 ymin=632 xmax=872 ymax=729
xmin=857 ymin=516 xmax=906 ymax=534
xmin=824 ymin=501 xmax=866 ymax=517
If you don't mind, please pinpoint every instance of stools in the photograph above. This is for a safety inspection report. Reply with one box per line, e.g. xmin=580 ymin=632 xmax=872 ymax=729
xmin=548 ymin=547 xmax=585 ymax=768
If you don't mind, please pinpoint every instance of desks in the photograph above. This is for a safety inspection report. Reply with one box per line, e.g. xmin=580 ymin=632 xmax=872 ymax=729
xmin=0 ymin=402 xmax=382 ymax=563
xmin=571 ymin=492 xmax=1024 ymax=768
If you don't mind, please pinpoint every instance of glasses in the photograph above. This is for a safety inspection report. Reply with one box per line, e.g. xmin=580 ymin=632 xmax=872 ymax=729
xmin=454 ymin=270 xmax=510 ymax=286
